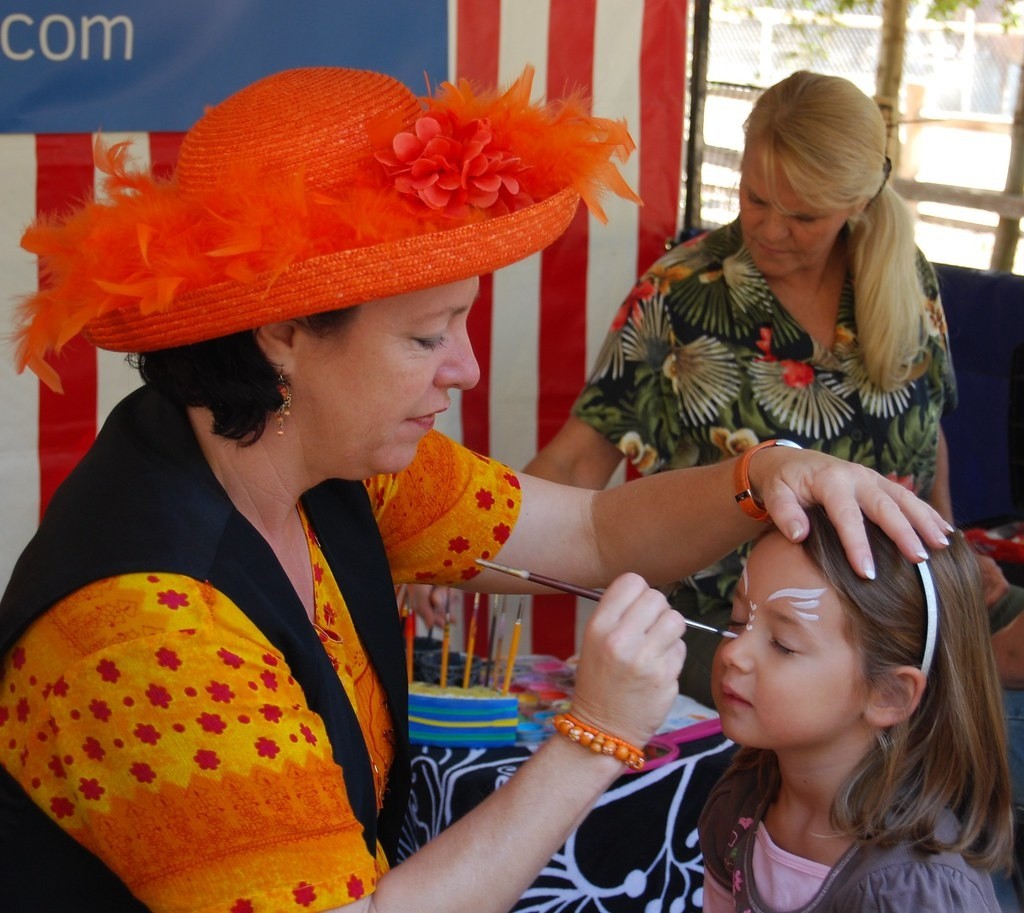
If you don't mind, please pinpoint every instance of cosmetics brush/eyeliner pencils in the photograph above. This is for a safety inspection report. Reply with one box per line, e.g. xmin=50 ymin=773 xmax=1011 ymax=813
xmin=475 ymin=559 xmax=739 ymax=638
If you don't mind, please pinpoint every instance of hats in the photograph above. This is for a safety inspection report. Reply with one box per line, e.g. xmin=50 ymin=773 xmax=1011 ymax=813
xmin=12 ymin=67 xmax=643 ymax=393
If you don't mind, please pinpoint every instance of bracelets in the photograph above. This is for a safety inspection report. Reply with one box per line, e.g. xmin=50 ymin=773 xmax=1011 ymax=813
xmin=552 ymin=713 xmax=645 ymax=770
xmin=733 ymin=439 xmax=802 ymax=524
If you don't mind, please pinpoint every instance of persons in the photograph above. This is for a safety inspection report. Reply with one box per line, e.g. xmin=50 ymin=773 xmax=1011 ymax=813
xmin=407 ymin=70 xmax=954 ymax=629
xmin=698 ymin=502 xmax=1013 ymax=913
xmin=0 ymin=69 xmax=956 ymax=913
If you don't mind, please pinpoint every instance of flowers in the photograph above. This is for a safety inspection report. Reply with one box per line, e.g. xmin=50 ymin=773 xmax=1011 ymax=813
xmin=376 ymin=111 xmax=529 ymax=215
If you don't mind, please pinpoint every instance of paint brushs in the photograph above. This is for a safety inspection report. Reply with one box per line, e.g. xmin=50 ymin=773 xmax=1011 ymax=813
xmin=473 ymin=557 xmax=738 ymax=639
xmin=403 ymin=584 xmax=526 ymax=696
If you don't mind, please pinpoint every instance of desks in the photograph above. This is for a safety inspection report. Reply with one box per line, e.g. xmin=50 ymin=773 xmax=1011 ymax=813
xmin=398 ymin=733 xmax=744 ymax=913
